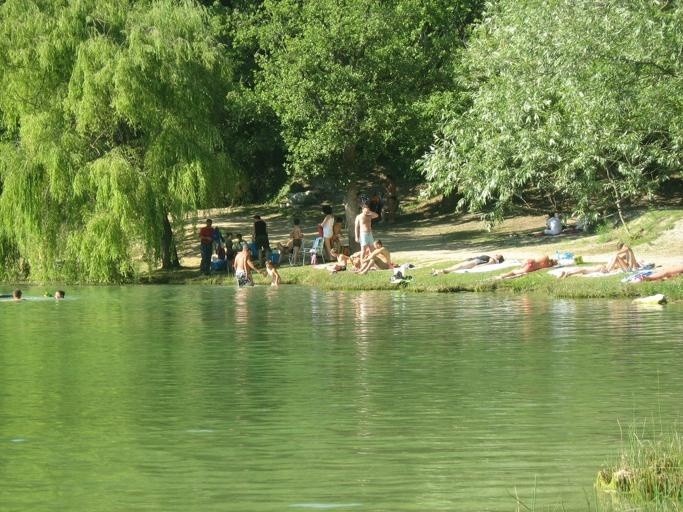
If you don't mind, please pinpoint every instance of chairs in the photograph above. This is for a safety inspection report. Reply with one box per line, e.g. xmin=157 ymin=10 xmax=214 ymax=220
xmin=277 ymin=236 xmax=325 ymax=266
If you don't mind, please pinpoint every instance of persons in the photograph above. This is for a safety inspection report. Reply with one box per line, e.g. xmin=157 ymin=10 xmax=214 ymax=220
xmin=543 ymin=206 xmax=567 ymax=234
xmin=199 ymin=205 xmax=390 ymax=287
xmin=493 ymin=255 xmax=559 ymax=279
xmin=13 ymin=289 xmax=22 ymax=299
xmin=602 ymin=241 xmax=638 ymax=272
xmin=55 ymin=290 xmax=65 ymax=299
xmin=431 ymin=254 xmax=504 ymax=274
